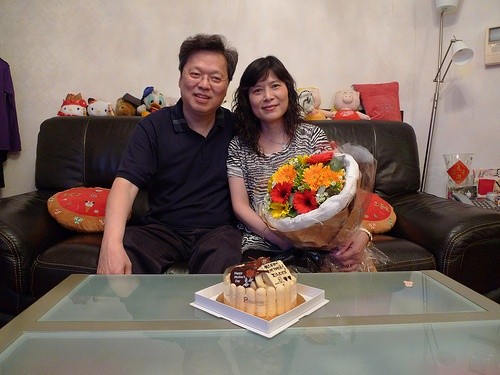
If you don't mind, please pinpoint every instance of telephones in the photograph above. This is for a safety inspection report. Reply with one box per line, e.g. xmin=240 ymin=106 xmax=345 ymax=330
xmin=449 ymin=192 xmax=500 ymax=211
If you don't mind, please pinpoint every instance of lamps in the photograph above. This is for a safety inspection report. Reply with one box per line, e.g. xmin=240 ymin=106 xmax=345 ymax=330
xmin=422 ymin=0 xmax=474 ymax=193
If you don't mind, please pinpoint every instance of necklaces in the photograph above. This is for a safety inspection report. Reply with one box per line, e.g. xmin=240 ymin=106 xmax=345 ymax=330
xmin=262 ymin=133 xmax=288 ymax=149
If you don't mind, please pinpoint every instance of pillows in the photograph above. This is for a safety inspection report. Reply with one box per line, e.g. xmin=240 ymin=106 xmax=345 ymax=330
xmin=48 ymin=187 xmax=132 ymax=233
xmin=361 ymin=193 xmax=398 ymax=233
xmin=353 ymin=82 xmax=402 ymax=122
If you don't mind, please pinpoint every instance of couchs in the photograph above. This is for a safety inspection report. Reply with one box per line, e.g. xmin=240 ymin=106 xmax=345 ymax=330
xmin=0 ymin=117 xmax=500 ymax=328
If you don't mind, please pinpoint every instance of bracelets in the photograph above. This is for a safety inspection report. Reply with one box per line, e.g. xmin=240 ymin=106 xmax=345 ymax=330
xmin=261 ymin=226 xmax=268 ymax=238
xmin=359 ymin=227 xmax=373 ymax=245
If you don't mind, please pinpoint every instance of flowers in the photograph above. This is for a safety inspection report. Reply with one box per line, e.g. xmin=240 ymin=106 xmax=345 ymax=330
xmin=267 ymin=149 xmax=347 ymax=219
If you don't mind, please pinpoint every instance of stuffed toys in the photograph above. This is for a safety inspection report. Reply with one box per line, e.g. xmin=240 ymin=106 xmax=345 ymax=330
xmin=137 ymin=86 xmax=168 ymax=117
xmin=88 ymin=98 xmax=114 ymax=117
xmin=327 ymin=90 xmax=371 ymax=121
xmin=57 ymin=93 xmax=87 ymax=118
xmin=109 ymin=92 xmax=137 ymax=116
xmin=295 ymin=86 xmax=335 ymax=120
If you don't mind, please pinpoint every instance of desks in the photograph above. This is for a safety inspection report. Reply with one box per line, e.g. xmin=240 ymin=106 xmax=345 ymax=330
xmin=0 ymin=271 xmax=500 ymax=375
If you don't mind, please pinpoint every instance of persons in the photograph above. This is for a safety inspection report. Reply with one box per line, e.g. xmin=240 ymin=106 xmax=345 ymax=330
xmin=98 ymin=35 xmax=244 ymax=274
xmin=108 ymin=274 xmax=366 ymax=375
xmin=227 ymin=55 xmax=377 ymax=274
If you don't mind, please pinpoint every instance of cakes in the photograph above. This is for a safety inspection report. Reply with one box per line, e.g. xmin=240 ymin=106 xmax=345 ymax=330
xmin=223 ymin=257 xmax=299 ymax=318
